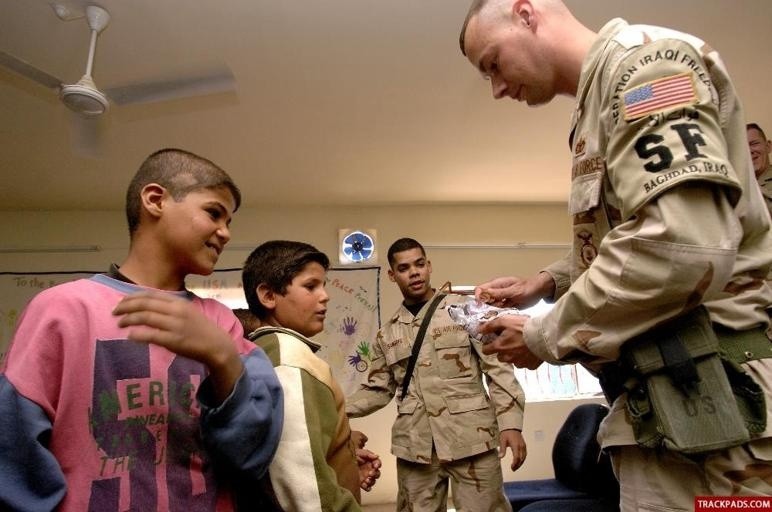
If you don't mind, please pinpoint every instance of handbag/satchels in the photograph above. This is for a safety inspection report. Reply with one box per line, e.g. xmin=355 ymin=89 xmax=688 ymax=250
xmin=629 ymin=313 xmax=751 ymax=456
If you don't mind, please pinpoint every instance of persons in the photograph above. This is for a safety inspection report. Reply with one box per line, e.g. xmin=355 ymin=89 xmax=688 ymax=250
xmin=345 ymin=237 xmax=527 ymax=512
xmin=0 ymin=149 xmax=283 ymax=512
xmin=746 ymin=123 xmax=772 ymax=215
xmin=235 ymin=308 xmax=368 ymax=449
xmin=460 ymin=0 xmax=772 ymax=512
xmin=245 ymin=241 xmax=382 ymax=512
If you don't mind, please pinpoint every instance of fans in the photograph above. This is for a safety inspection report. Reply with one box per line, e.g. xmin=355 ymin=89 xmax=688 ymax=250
xmin=0 ymin=6 xmax=237 ymax=158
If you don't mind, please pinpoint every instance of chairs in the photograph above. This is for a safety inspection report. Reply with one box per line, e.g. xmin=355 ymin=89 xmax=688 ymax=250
xmin=519 ymin=460 xmax=619 ymax=511
xmin=503 ymin=404 xmax=608 ymax=506
